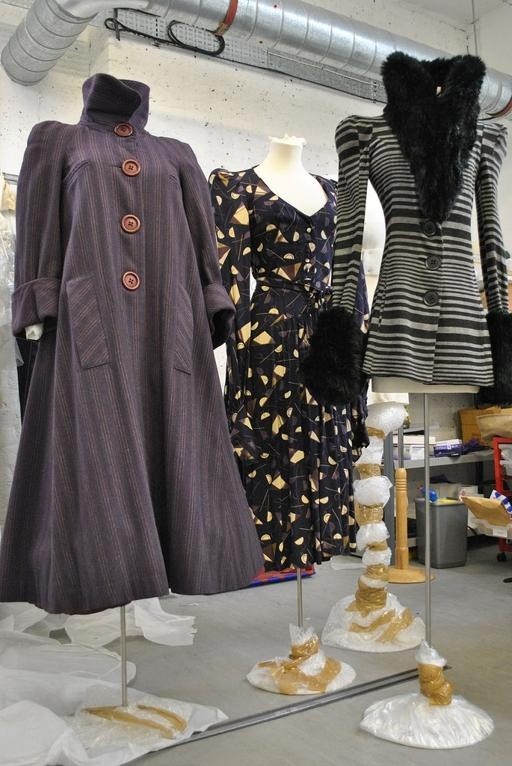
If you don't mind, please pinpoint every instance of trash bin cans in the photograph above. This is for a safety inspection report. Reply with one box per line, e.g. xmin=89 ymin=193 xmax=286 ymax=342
xmin=415 ymin=495 xmax=468 ymax=569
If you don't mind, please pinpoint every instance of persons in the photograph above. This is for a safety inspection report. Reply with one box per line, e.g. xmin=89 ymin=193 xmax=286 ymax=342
xmin=197 ymin=131 xmax=374 ymax=575
xmin=296 ymin=47 xmax=512 ymax=420
xmin=2 ymin=69 xmax=268 ymax=620
